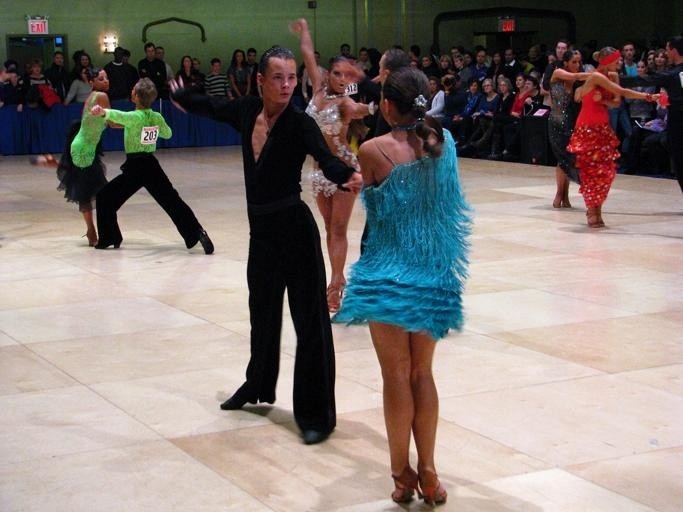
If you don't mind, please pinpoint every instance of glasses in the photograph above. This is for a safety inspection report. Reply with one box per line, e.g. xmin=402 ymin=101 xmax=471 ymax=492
xmin=483 ymin=83 xmax=492 ymax=88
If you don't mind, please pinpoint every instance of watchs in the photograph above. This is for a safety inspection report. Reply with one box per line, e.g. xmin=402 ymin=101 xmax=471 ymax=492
xmin=445 ymin=91 xmax=449 ymax=93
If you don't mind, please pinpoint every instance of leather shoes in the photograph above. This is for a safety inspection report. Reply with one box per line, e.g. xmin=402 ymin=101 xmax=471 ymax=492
xmin=201 ymin=230 xmax=215 ymax=254
xmin=93 ymin=236 xmax=122 ymax=249
xmin=221 ymin=391 xmax=248 ymax=409
xmin=302 ymin=429 xmax=327 ymax=443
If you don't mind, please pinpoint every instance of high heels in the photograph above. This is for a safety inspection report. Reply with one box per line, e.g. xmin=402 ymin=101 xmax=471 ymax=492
xmin=562 ymin=200 xmax=571 ymax=207
xmin=553 ymin=192 xmax=563 ymax=208
xmin=31 ymin=153 xmax=58 ymax=166
xmin=339 ymin=279 xmax=347 ymax=297
xmin=418 ymin=467 xmax=448 ymax=507
xmin=587 ymin=211 xmax=600 ymax=228
xmin=327 ymin=283 xmax=341 ymax=311
xmin=392 ymin=463 xmax=423 ymax=501
xmin=87 ymin=228 xmax=98 ymax=248
xmin=598 ymin=213 xmax=605 ymax=228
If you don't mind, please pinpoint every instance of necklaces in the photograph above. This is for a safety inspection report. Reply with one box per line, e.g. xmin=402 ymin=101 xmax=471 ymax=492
xmin=322 ymin=85 xmax=341 ymax=103
xmin=395 ymin=123 xmax=422 ymax=135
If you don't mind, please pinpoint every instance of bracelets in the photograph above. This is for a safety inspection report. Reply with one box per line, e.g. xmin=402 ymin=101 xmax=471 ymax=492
xmin=647 ymin=92 xmax=652 ymax=103
xmin=369 ymin=100 xmax=375 ymax=116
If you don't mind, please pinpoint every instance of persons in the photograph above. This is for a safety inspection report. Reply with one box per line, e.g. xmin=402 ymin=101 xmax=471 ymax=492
xmin=543 ymin=41 xmax=585 ymax=90
xmin=441 ymin=73 xmax=468 ymax=136
xmin=248 ymin=47 xmax=259 ymax=96
xmin=30 ymin=66 xmax=125 ymax=246
xmin=330 ymin=68 xmax=475 ymax=506
xmin=608 ymin=54 xmax=633 ymax=175
xmin=540 ymin=52 xmax=557 ymax=165
xmin=192 ymin=58 xmax=199 ymax=69
xmin=27 ymin=59 xmax=53 ymax=107
xmin=91 ymin=77 xmax=215 ymax=255
xmin=137 ymin=43 xmax=166 ymax=91
xmin=647 ymin=51 xmax=656 ymax=67
xmin=357 ymin=47 xmax=368 ymax=69
xmin=567 ymin=47 xmax=661 ymax=227
xmin=154 ymin=47 xmax=174 ymax=80
xmin=549 ymin=50 xmax=593 ymax=208
xmin=503 ymin=75 xmax=529 ymax=160
xmin=522 ymin=77 xmax=544 ymax=162
xmin=623 ymin=36 xmax=683 ymax=193
xmin=62 ymin=50 xmax=94 ymax=105
xmin=168 ymin=44 xmax=363 ymax=444
xmin=575 ymin=50 xmax=596 ymax=72
xmin=367 ymin=48 xmax=409 ymax=136
xmin=423 ymin=76 xmax=445 ymax=123
xmin=46 ymin=51 xmax=70 ymax=101
xmin=405 ymin=43 xmax=543 ymax=82
xmin=228 ymin=49 xmax=251 ymax=98
xmin=460 ymin=79 xmax=500 ymax=155
xmin=654 ymin=49 xmax=667 ymax=71
xmin=364 ymin=48 xmax=383 ymax=78
xmin=453 ymin=79 xmax=482 ymax=158
xmin=104 ymin=46 xmax=138 ymax=101
xmin=303 ymin=51 xmax=328 ymax=100
xmin=205 ymin=58 xmax=235 ymax=100
xmin=176 ymin=55 xmax=203 ymax=89
xmin=626 ymin=59 xmax=656 ymax=127
xmin=622 ymin=42 xmax=638 ymax=103
xmin=341 ymin=44 xmax=355 ymax=60
xmin=471 ymin=78 xmax=515 ymax=159
xmin=0 ymin=57 xmax=27 ymax=111
xmin=289 ymin=18 xmax=379 ymax=312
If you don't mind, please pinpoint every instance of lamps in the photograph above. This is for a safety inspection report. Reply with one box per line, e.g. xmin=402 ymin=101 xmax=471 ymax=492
xmin=102 ymin=35 xmax=118 ymax=52
